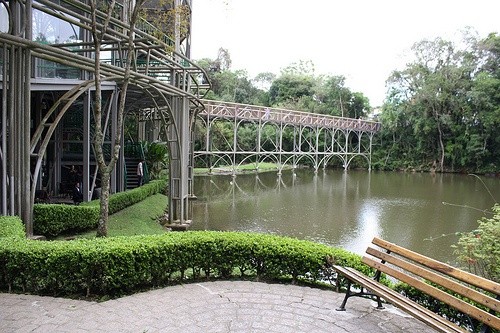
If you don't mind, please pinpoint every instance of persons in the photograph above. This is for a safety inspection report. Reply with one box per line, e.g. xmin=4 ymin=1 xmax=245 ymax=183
xmin=137 ymin=158 xmax=145 ymax=187
xmin=73 ymin=182 xmax=82 ymax=205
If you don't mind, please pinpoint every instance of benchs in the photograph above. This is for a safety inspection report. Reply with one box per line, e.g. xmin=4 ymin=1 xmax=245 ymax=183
xmin=331 ymin=236 xmax=500 ymax=333
